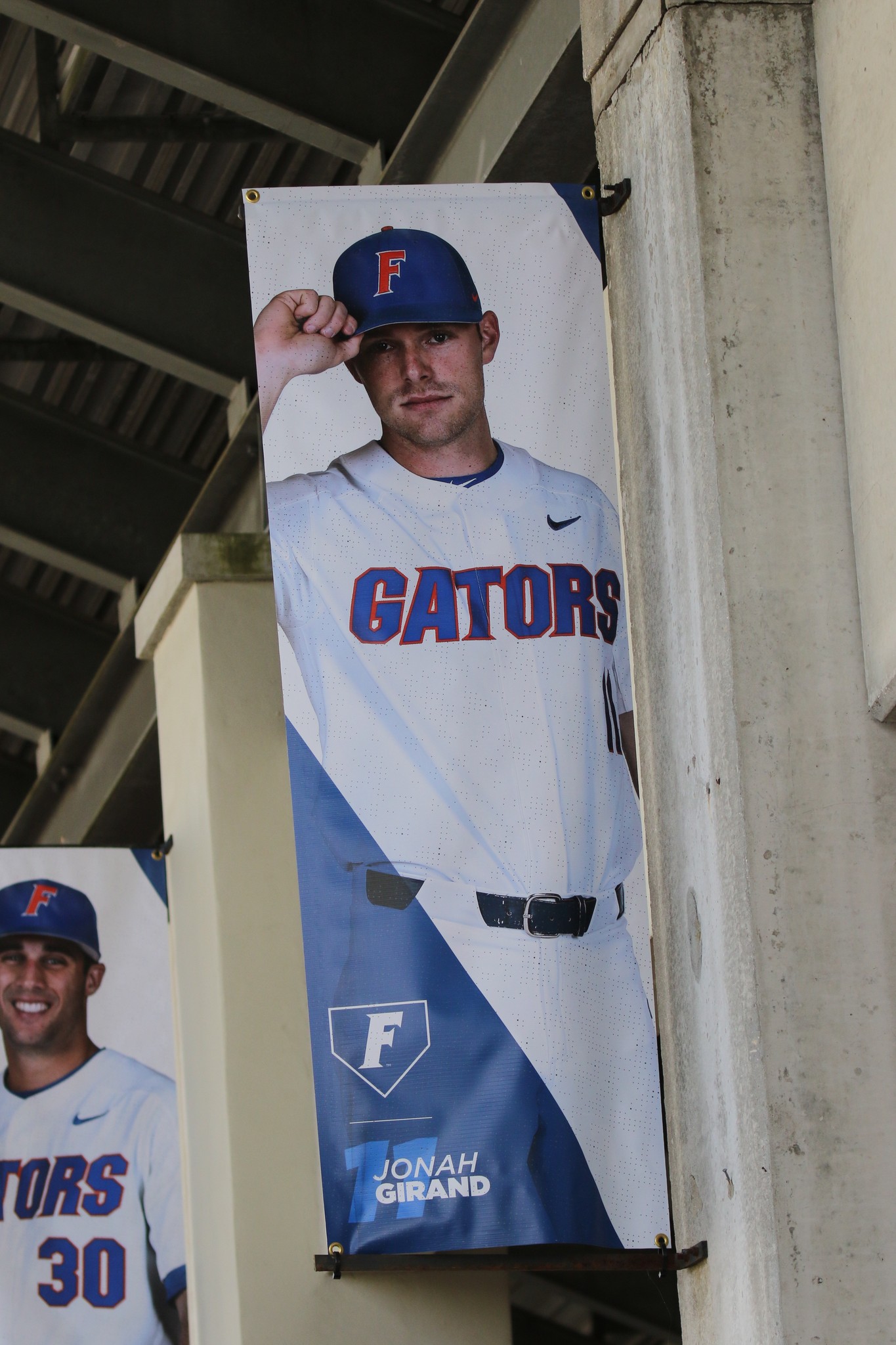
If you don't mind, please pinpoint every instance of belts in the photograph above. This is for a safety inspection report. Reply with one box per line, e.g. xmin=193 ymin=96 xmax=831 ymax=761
xmin=366 ymin=869 xmax=626 ymax=938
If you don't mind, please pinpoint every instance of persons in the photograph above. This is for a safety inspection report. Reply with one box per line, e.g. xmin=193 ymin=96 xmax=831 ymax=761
xmin=251 ymin=225 xmax=671 ymax=1251
xmin=0 ymin=880 xmax=190 ymax=1345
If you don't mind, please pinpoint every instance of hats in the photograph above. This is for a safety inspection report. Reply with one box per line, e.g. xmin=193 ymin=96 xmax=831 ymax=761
xmin=333 ymin=227 xmax=483 ymax=342
xmin=0 ymin=880 xmax=101 ymax=963
